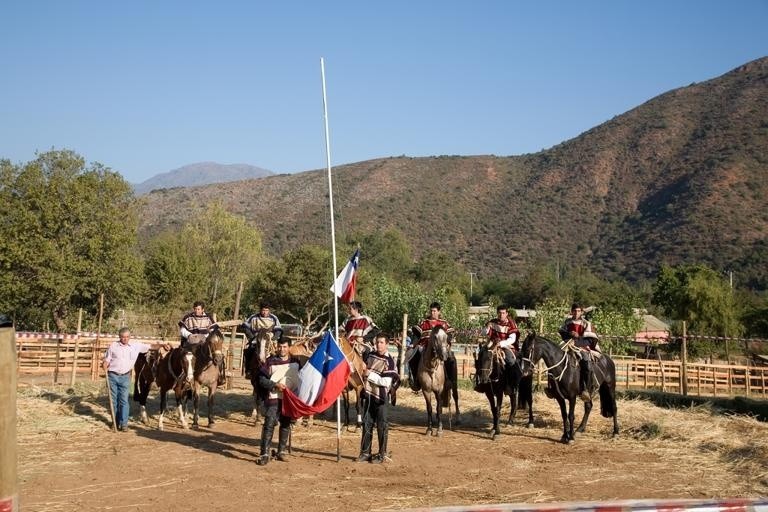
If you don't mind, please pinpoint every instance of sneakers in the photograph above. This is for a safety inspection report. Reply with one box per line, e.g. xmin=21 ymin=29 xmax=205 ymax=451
xmin=355 ymin=454 xmax=372 ymax=461
xmin=371 ymin=454 xmax=384 ymax=463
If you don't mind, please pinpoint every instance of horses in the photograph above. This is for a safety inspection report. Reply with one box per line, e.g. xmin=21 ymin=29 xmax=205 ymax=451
xmin=473 ymin=339 xmax=536 ymax=441
xmin=133 ymin=344 xmax=195 ymax=431
xmin=181 ymin=326 xmax=226 ymax=428
xmin=415 ymin=326 xmax=463 ymax=438
xmin=243 ymin=324 xmax=403 ymax=425
xmin=519 ymin=331 xmax=620 ymax=447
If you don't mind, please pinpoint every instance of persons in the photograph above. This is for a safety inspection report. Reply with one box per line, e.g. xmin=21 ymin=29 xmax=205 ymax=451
xmin=102 ymin=328 xmax=171 ymax=432
xmin=242 ymin=301 xmax=281 ymax=380
xmin=402 ymin=302 xmax=456 ymax=392
xmin=338 ymin=301 xmax=380 ymax=355
xmin=472 ymin=305 xmax=525 ymax=394
xmin=256 ymin=337 xmax=303 ymax=466
xmin=178 ymin=301 xmax=221 ymax=352
xmin=544 ymin=304 xmax=602 ymax=402
xmin=352 ymin=333 xmax=401 ymax=463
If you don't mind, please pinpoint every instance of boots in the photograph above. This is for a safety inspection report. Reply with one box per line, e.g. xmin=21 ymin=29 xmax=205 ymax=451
xmin=257 ymin=423 xmax=276 ymax=465
xmin=277 ymin=426 xmax=290 ymax=461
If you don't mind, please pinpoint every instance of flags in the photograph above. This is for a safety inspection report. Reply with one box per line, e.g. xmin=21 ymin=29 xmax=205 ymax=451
xmin=281 ymin=329 xmax=353 ymax=419
xmin=330 ymin=248 xmax=359 ymax=302
